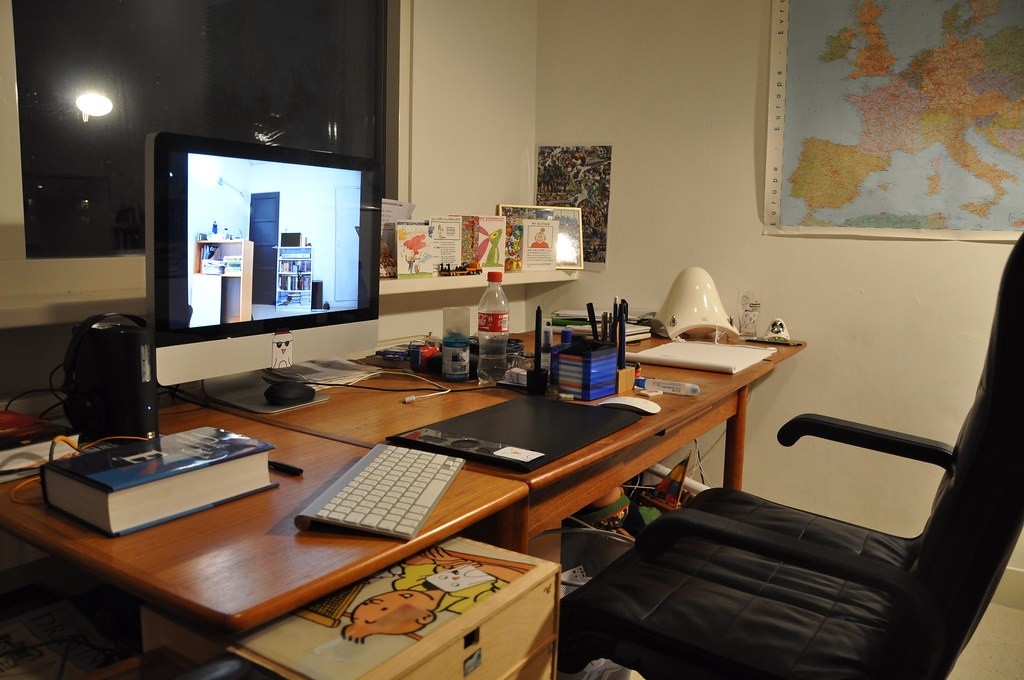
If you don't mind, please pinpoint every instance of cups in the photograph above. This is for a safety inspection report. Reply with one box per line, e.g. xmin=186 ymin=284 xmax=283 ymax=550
xmin=441 ymin=307 xmax=470 ymax=381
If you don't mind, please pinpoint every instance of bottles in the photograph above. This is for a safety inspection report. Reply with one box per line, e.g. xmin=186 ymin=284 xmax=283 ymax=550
xmin=551 ymin=329 xmax=573 ymax=386
xmin=477 ymin=271 xmax=511 ymax=385
xmin=541 ymin=327 xmax=553 ymax=375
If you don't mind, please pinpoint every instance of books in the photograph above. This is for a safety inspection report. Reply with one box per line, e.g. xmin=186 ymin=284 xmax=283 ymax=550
xmin=0 ymin=410 xmax=80 ymax=484
xmin=39 ymin=426 xmax=279 ymax=538
xmin=277 ymin=260 xmax=311 ymax=306
xmin=550 ymin=309 xmax=652 ymax=343
xmin=199 ymin=245 xmax=241 ymax=275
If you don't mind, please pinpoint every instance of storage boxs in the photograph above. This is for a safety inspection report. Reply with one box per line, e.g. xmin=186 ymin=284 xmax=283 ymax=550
xmin=561 ymin=484 xmax=695 ymax=578
xmin=559 ymin=340 xmax=618 ymax=401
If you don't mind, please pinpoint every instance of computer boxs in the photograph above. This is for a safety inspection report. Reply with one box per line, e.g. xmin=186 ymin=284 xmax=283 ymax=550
xmin=70 ymin=321 xmax=158 ymax=443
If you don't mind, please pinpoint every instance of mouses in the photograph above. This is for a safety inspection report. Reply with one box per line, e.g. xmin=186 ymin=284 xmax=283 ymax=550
xmin=263 ymin=381 xmax=315 ymax=406
xmin=598 ymin=396 xmax=661 ymax=415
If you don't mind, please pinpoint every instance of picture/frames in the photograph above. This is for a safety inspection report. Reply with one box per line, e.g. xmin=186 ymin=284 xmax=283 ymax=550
xmin=498 ymin=204 xmax=584 ymax=269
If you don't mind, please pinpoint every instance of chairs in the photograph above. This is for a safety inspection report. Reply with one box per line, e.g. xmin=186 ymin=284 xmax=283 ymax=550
xmin=557 ymin=234 xmax=1024 ymax=680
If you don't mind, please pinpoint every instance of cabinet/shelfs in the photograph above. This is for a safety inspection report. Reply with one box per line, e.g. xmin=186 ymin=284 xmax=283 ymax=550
xmin=276 ymin=247 xmax=311 ymax=312
xmin=140 ymin=537 xmax=561 ymax=680
xmin=194 ymin=240 xmax=254 ymax=324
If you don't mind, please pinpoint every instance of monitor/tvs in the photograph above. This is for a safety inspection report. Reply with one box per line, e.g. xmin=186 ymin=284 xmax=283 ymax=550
xmin=144 ymin=132 xmax=379 ymax=414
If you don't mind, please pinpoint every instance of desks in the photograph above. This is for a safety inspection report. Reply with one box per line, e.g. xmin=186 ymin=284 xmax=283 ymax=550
xmin=0 ymin=331 xmax=807 ymax=631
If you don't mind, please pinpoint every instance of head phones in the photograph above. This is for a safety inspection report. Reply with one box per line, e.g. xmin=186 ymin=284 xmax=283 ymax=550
xmin=64 ymin=312 xmax=147 ymax=428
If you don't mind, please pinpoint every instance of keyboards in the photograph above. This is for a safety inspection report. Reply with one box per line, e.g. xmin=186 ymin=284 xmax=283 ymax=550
xmin=294 ymin=443 xmax=465 ymax=540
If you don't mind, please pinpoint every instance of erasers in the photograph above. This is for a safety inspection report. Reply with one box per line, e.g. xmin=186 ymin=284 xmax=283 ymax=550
xmin=558 ymin=393 xmax=575 ymax=401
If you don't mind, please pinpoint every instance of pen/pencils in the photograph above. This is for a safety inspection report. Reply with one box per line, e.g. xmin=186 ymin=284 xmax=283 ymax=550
xmin=619 ymin=295 xmax=629 ymax=371
xmin=586 ymin=302 xmax=599 ymax=340
xmin=268 ymin=459 xmax=304 ymax=476
xmin=608 ymin=312 xmax=613 ymax=342
xmin=613 ymin=296 xmax=618 ymax=343
xmin=740 ymin=339 xmax=803 ymax=346
xmin=601 ymin=312 xmax=608 ymax=341
